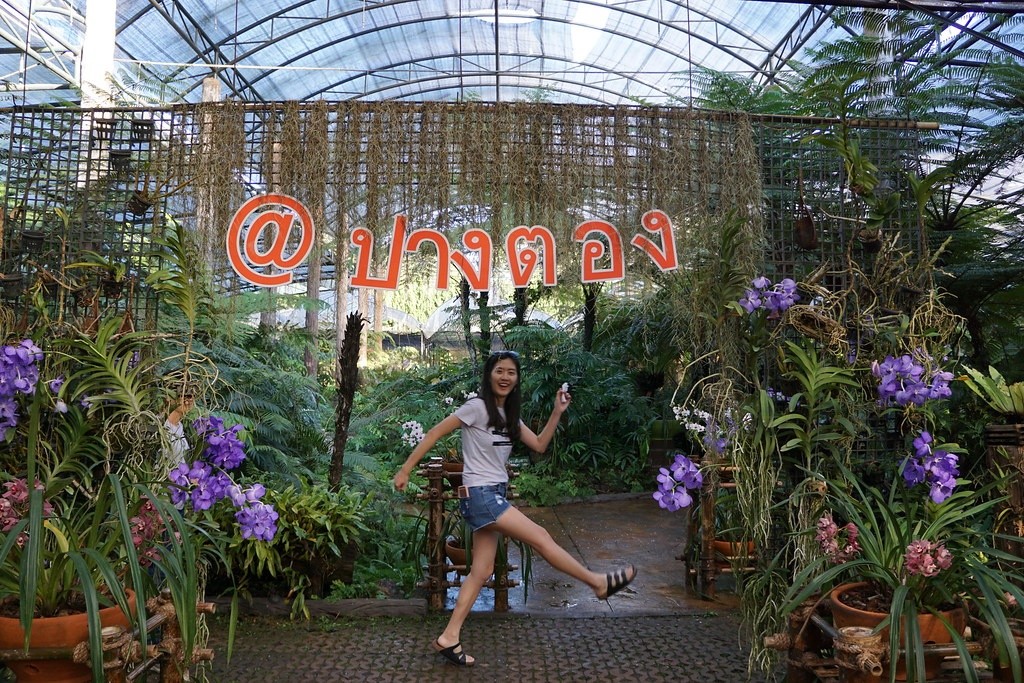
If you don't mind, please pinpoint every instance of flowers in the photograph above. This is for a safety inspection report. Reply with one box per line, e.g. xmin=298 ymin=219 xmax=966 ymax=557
xmin=0 ymin=340 xmax=279 ymax=683
xmin=652 ymin=277 xmax=1024 ymax=683
xmin=401 ymin=389 xmax=478 ymax=462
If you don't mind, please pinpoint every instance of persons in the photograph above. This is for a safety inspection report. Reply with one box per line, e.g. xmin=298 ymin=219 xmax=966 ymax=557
xmin=117 ymin=396 xmax=193 ymax=674
xmin=393 ymin=350 xmax=638 ymax=667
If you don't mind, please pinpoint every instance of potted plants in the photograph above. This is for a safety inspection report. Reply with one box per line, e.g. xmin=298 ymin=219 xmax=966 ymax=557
xmin=128 ymin=170 xmax=201 ymax=217
xmin=857 ymin=227 xmax=883 ymax=254
xmin=402 ymin=501 xmax=534 ymax=603
xmin=26 ymin=260 xmax=74 ymax=304
xmin=63 ymin=249 xmax=129 ymax=298
xmin=68 ymin=277 xmax=95 ymax=308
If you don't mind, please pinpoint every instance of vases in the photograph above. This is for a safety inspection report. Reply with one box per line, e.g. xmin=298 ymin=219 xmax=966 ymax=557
xmin=21 ymin=231 xmax=45 ymax=255
xmin=831 ymin=581 xmax=969 ymax=680
xmin=96 ymin=119 xmax=118 ymax=141
xmin=1 ymin=274 xmax=25 ymax=297
xmin=132 ymin=119 xmax=155 ymax=143
xmin=440 ymin=456 xmax=464 ymax=493
xmin=713 ymin=540 xmax=754 ymax=557
xmin=0 ymin=589 xmax=138 ymax=683
xmin=108 ymin=151 xmax=131 ymax=173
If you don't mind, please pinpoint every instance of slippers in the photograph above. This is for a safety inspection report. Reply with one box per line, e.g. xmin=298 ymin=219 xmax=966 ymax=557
xmin=431 ymin=638 xmax=476 ymax=668
xmin=597 ymin=564 xmax=637 ymax=601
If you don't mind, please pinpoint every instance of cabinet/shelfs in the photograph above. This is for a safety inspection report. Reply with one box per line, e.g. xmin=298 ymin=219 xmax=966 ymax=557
xmin=415 ymin=456 xmax=520 ymax=611
